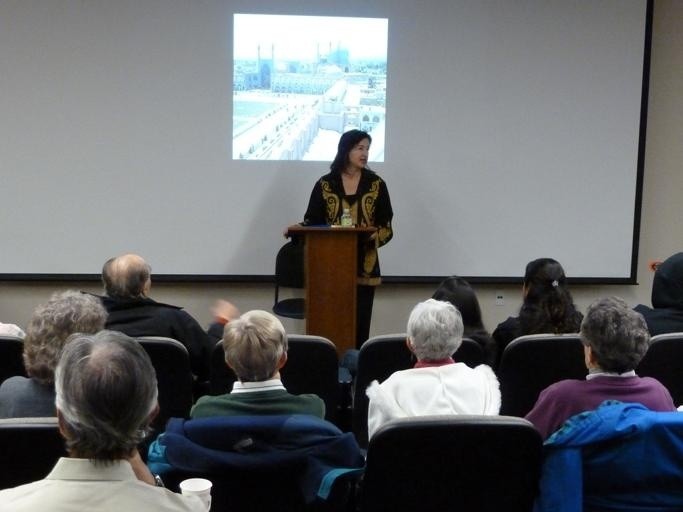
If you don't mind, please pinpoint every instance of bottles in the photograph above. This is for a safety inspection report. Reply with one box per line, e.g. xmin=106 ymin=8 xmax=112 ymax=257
xmin=341 ymin=208 xmax=354 ymax=227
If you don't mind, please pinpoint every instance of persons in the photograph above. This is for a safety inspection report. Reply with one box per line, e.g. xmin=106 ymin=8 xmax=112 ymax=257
xmin=489 ymin=254 xmax=582 ymax=353
xmin=0 ymin=321 xmax=25 ymax=343
xmin=0 ymin=328 xmax=207 ymax=512
xmin=93 ymin=252 xmax=240 ymax=397
xmin=362 ymin=292 xmax=503 ymax=447
xmin=283 ymin=128 xmax=394 ymax=350
xmin=630 ymin=248 xmax=683 ymax=340
xmin=182 ymin=306 xmax=329 ymax=420
xmin=0 ymin=288 xmax=111 ymax=420
xmin=431 ymin=274 xmax=495 ymax=360
xmin=517 ymin=293 xmax=677 ymax=446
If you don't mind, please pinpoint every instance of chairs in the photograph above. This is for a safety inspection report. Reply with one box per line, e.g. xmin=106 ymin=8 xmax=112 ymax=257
xmin=273 ymin=240 xmax=306 ymax=320
xmin=1 ymin=332 xmax=681 ymax=512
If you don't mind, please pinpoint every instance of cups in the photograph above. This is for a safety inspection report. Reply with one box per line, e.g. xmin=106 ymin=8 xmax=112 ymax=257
xmin=179 ymin=476 xmax=212 ymax=510
